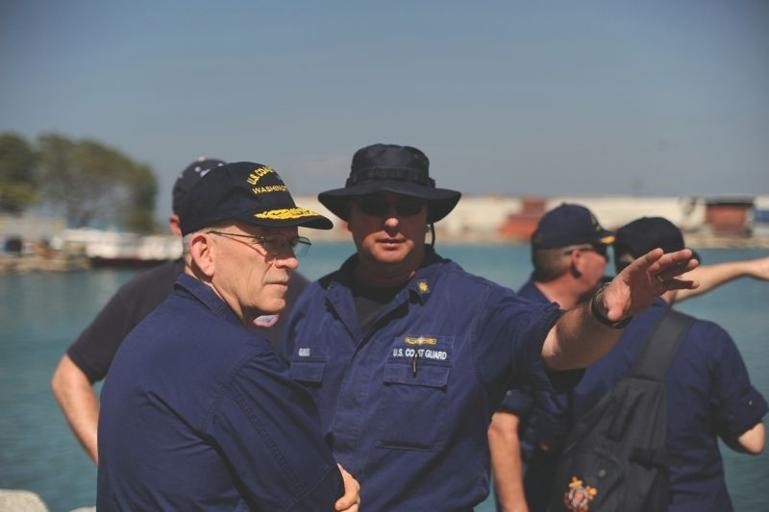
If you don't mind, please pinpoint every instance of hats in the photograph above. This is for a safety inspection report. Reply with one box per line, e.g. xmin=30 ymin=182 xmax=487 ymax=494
xmin=319 ymin=144 xmax=461 ymax=223
xmin=182 ymin=162 xmax=332 ymax=236
xmin=173 ymin=159 xmax=225 ymax=216
xmin=532 ymin=205 xmax=616 ymax=250
xmin=615 ymin=217 xmax=699 ymax=275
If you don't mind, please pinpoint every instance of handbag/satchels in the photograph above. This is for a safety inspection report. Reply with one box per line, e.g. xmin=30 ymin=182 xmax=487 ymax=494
xmin=524 ymin=377 xmax=673 ymax=511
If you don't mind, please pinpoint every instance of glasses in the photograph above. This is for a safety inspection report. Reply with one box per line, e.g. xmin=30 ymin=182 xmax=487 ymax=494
xmin=564 ymin=243 xmax=606 ymax=254
xmin=357 ymin=198 xmax=422 ymax=216
xmin=204 ymin=230 xmax=311 ymax=259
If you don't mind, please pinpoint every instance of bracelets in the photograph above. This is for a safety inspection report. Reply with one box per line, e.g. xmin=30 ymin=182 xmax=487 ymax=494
xmin=588 ymin=280 xmax=633 ymax=329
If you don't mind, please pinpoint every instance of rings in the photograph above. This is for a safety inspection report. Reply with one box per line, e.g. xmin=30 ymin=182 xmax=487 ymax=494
xmin=654 ymin=273 xmax=666 ymax=284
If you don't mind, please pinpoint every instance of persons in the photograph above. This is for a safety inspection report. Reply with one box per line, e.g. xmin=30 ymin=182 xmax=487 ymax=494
xmin=49 ymin=156 xmax=317 ymax=467
xmin=564 ymin=215 xmax=769 ymax=511
xmin=95 ymin=160 xmax=363 ymax=511
xmin=280 ymin=142 xmax=702 ymax=511
xmin=482 ymin=199 xmax=769 ymax=511
